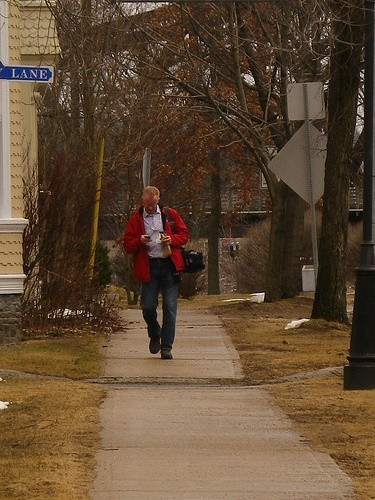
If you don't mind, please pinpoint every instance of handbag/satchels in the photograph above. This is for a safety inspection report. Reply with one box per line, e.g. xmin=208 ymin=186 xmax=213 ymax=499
xmin=181 ymin=248 xmax=205 ymax=274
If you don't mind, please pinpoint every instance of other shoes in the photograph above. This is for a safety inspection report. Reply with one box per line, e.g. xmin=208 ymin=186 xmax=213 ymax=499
xmin=160 ymin=349 xmax=173 ymax=360
xmin=149 ymin=338 xmax=160 ymax=354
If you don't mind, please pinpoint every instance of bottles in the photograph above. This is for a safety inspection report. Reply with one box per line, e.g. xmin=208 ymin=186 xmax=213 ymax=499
xmin=160 ymin=233 xmax=171 ymax=257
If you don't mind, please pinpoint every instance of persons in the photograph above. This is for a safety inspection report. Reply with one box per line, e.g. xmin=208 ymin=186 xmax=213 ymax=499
xmin=124 ymin=186 xmax=189 ymax=360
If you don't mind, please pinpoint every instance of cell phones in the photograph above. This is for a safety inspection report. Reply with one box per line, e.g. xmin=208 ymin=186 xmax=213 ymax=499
xmin=145 ymin=236 xmax=150 ymax=242
